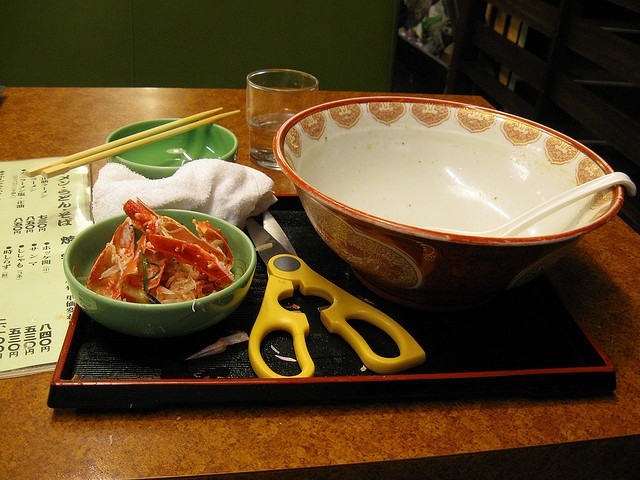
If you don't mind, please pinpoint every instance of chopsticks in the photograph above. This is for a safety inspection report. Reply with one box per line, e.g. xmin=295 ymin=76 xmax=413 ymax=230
xmin=25 ymin=106 xmax=242 ymax=179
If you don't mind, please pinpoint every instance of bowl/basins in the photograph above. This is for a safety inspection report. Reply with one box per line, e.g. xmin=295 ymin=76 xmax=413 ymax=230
xmin=63 ymin=209 xmax=258 ymax=340
xmin=272 ymin=95 xmax=624 ymax=321
xmin=104 ymin=118 xmax=238 ymax=180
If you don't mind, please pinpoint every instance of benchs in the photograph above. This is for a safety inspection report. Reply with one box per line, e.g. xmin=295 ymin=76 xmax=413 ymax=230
xmin=441 ymin=0 xmax=639 ymax=237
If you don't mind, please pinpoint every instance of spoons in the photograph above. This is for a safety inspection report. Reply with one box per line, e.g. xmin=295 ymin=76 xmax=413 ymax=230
xmin=416 ymin=171 xmax=636 ymax=238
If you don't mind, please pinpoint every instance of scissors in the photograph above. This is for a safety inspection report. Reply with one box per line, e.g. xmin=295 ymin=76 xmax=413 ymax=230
xmin=242 ymin=210 xmax=430 ymax=378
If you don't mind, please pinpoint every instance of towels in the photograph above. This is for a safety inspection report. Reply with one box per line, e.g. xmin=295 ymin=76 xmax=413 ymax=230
xmin=90 ymin=158 xmax=277 ymax=230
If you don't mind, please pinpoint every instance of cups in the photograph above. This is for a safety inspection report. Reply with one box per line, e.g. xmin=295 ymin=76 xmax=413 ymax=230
xmin=245 ymin=68 xmax=319 ymax=172
